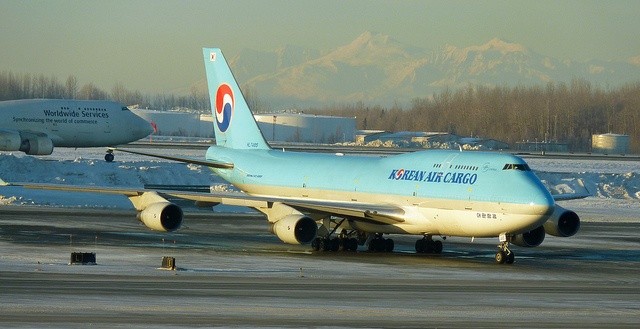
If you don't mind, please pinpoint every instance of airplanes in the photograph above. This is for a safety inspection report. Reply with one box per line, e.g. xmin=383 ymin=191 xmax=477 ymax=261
xmin=0 ymin=47 xmax=593 ymax=263
xmin=0 ymin=98 xmax=154 ymax=160
